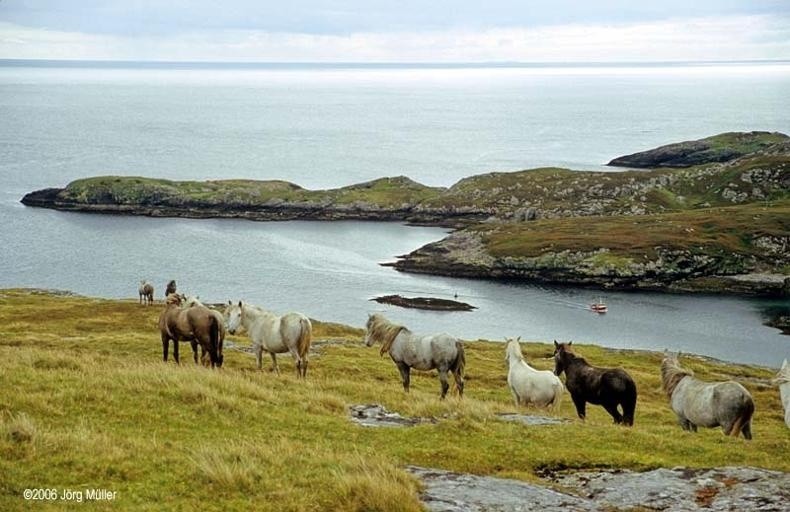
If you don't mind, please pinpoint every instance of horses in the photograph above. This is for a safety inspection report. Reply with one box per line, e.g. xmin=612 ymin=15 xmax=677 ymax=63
xmin=364 ymin=312 xmax=466 ymax=402
xmin=223 ymin=300 xmax=312 ymax=380
xmin=158 ymin=293 xmax=225 ymax=370
xmin=553 ymin=339 xmax=637 ymax=427
xmin=769 ymin=358 xmax=790 ymax=429
xmin=504 ymin=335 xmax=565 ymax=415
xmin=659 ymin=348 xmax=755 ymax=443
xmin=138 ymin=280 xmax=154 ymax=306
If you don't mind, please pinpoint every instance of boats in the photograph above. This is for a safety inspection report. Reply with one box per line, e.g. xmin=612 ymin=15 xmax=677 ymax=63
xmin=589 ymin=301 xmax=608 ymax=313
